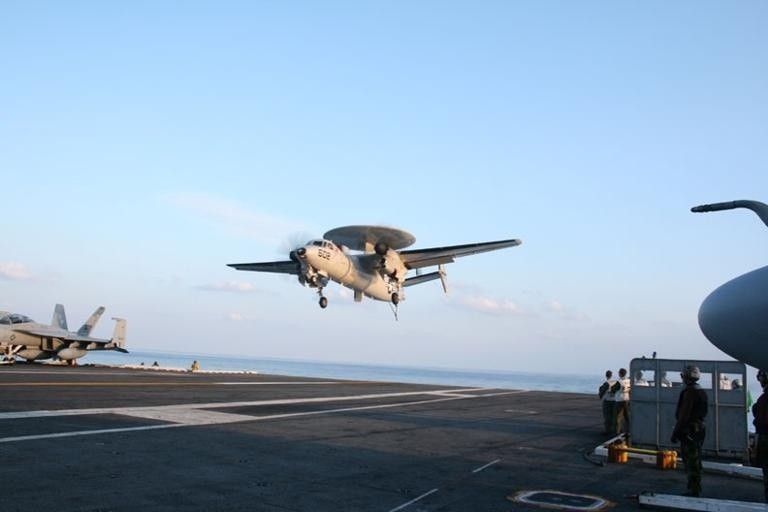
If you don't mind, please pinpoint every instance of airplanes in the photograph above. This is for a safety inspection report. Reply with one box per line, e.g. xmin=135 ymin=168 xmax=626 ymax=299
xmin=0 ymin=301 xmax=131 ymax=369
xmin=690 ymin=198 xmax=768 ymax=376
xmin=225 ymin=225 xmax=523 ymax=321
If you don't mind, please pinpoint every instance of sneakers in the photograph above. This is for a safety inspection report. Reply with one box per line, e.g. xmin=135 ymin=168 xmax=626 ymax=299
xmin=681 ymin=483 xmax=704 ymax=497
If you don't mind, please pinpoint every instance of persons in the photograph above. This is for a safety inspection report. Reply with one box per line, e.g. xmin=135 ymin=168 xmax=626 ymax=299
xmin=729 ymin=379 xmax=740 ymax=389
xmin=633 ymin=371 xmax=649 ymax=386
xmin=610 ymin=368 xmax=632 ymax=434
xmin=600 ymin=369 xmax=618 ymax=437
xmin=654 ymin=371 xmax=672 ymax=387
xmin=670 ymin=363 xmax=709 ymax=497
xmin=192 ymin=360 xmax=201 ymax=371
xmin=753 ymin=368 xmax=767 ymax=504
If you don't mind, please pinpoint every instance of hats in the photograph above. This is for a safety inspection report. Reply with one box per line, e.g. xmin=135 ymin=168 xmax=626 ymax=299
xmin=606 ymin=368 xmax=627 ymax=377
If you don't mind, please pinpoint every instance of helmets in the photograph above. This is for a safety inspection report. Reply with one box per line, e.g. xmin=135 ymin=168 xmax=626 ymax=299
xmin=682 ymin=365 xmax=700 ymax=380
